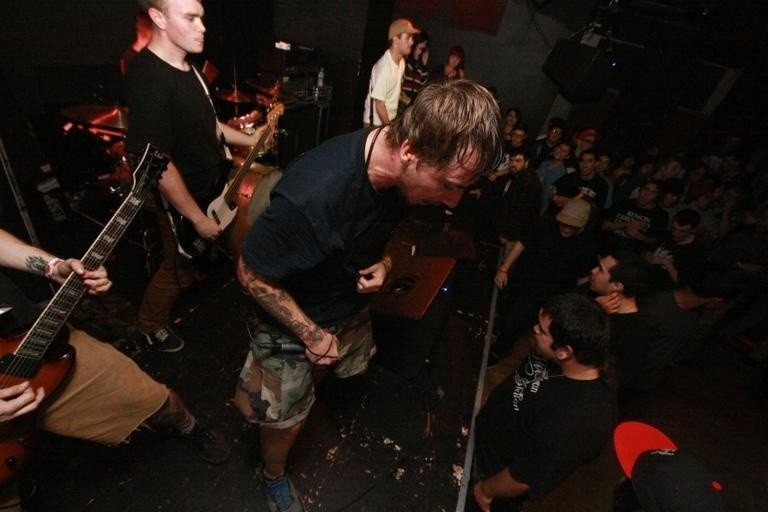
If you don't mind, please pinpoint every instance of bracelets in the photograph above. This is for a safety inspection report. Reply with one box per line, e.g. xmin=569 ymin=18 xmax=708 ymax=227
xmin=45 ymin=257 xmax=65 ymax=280
xmin=381 ymin=255 xmax=394 ymax=272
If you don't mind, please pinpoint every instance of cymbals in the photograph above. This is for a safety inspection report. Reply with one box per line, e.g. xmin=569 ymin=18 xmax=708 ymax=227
xmin=61 ymin=106 xmax=130 ymax=128
xmin=215 ymin=90 xmax=254 ymax=102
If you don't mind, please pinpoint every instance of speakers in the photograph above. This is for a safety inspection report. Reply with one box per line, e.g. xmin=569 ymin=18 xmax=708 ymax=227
xmin=542 ymin=36 xmax=606 ymax=106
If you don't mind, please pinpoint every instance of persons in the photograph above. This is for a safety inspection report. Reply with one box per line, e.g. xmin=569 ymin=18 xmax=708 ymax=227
xmin=0 ymin=229 xmax=231 ymax=512
xmin=397 ymin=33 xmax=768 ymax=511
xmin=234 ymin=80 xmax=504 ymax=511
xmin=363 ymin=18 xmax=420 ymax=131
xmin=127 ymin=1 xmax=274 ymax=352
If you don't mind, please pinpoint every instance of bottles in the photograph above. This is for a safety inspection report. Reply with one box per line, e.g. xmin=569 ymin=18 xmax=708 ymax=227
xmin=316 ymin=67 xmax=325 ymax=87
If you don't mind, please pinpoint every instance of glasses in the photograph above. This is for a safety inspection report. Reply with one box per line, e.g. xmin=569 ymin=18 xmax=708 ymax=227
xmin=511 ymin=133 xmax=524 ymax=138
xmin=552 ymin=129 xmax=560 ymax=136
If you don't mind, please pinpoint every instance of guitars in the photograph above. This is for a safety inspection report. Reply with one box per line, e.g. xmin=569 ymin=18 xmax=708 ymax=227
xmin=175 ymin=102 xmax=285 ymax=257
xmin=0 ymin=142 xmax=170 ymax=485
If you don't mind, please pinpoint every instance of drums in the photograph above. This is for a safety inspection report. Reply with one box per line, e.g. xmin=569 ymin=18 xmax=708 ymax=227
xmin=217 ymin=154 xmax=285 ymax=261
xmin=227 ymin=110 xmax=278 ymax=158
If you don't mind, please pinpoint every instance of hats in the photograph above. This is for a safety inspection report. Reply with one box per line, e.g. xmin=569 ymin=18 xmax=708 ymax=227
xmin=388 ymin=19 xmax=421 ymax=40
xmin=555 ymin=199 xmax=591 ymax=228
xmin=450 ymin=46 xmax=464 ymax=59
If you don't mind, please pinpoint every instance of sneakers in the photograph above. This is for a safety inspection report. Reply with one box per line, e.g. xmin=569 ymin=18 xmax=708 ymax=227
xmin=261 ymin=467 xmax=305 ymax=512
xmin=138 ymin=327 xmax=185 ymax=352
xmin=193 ymin=427 xmax=230 ymax=465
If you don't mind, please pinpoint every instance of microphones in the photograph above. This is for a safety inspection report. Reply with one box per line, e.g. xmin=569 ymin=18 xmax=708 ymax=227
xmin=271 ymin=334 xmax=342 ymax=353
